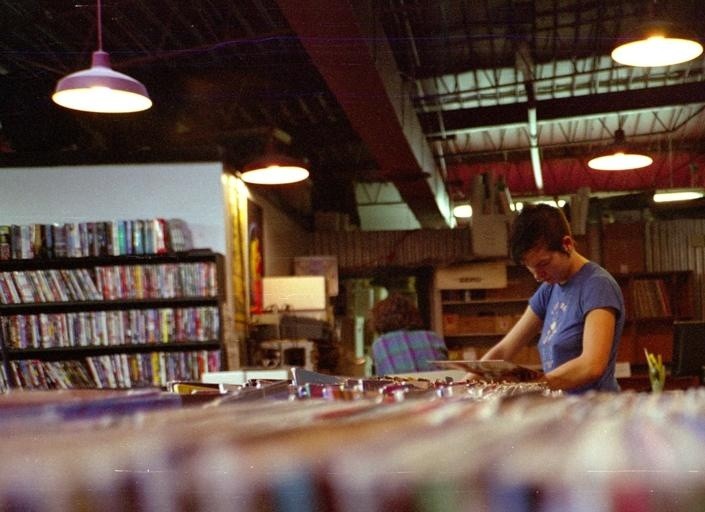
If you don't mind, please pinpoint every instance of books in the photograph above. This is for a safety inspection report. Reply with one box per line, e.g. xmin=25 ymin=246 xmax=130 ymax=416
xmin=444 ymin=221 xmax=704 ymax=365
xmin=670 ymin=323 xmax=704 ymax=378
xmin=469 ymin=176 xmax=513 ymax=213
xmin=1 ymin=365 xmax=705 ymax=511
xmin=2 ymin=219 xmax=221 ymax=390
xmin=570 ymin=185 xmax=591 ymax=236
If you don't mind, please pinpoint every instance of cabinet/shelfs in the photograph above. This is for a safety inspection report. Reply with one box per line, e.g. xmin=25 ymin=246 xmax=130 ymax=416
xmin=594 ymin=216 xmax=705 ymax=391
xmin=428 ymin=249 xmax=541 ymax=374
xmin=1 ymin=244 xmax=228 ymax=393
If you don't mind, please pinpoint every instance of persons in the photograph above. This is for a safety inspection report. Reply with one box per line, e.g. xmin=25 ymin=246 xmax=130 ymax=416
xmin=460 ymin=201 xmax=627 ymax=394
xmin=369 ymin=294 xmax=452 ymax=377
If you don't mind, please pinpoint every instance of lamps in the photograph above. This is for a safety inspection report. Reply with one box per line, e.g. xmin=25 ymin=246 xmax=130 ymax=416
xmin=237 ymin=22 xmax=313 ymax=189
xmin=49 ymin=0 xmax=157 ymax=115
xmin=586 ymin=117 xmax=654 ymax=171
xmin=609 ymin=0 xmax=704 ymax=72
